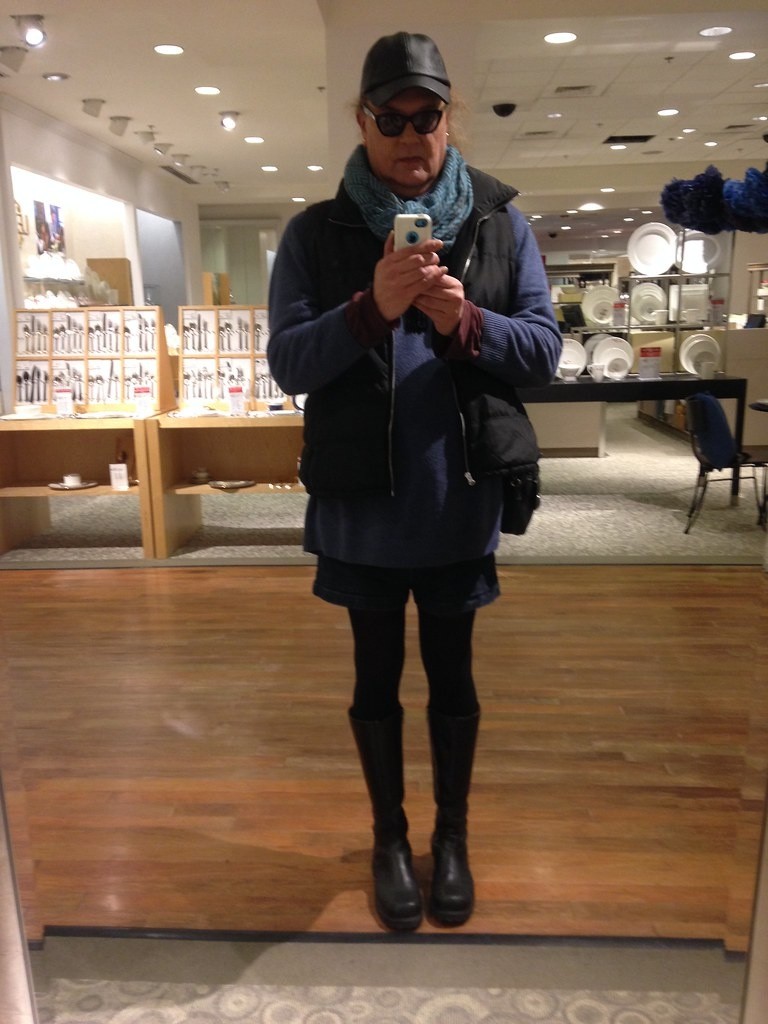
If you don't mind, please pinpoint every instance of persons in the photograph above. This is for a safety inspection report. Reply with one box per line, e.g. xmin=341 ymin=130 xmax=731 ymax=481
xmin=266 ymin=33 xmax=562 ymax=937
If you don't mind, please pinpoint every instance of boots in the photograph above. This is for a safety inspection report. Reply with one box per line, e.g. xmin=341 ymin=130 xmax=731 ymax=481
xmin=429 ymin=702 xmax=482 ymax=927
xmin=347 ymin=703 xmax=422 ymax=931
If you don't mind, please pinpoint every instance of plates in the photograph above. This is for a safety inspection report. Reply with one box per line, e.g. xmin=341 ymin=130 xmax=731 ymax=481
xmin=47 ymin=478 xmax=98 ymax=490
xmin=208 ymin=480 xmax=256 ymax=488
xmin=556 ymin=222 xmax=723 ymax=376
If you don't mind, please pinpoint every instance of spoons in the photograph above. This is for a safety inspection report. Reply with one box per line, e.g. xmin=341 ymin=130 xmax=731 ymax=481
xmin=23 ymin=324 xmax=29 ymax=351
xmin=16 ymin=372 xmax=28 ymax=402
xmin=183 ymin=323 xmax=284 ymax=400
xmin=53 ymin=320 xmax=154 ymax=400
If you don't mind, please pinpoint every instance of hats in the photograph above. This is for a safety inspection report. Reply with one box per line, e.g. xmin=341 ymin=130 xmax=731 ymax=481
xmin=360 ymin=31 xmax=452 ymax=107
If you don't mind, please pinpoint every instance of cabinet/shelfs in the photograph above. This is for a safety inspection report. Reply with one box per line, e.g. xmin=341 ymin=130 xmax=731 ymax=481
xmin=0 ymin=305 xmax=306 ymax=560
xmin=570 ymin=225 xmax=736 ymax=373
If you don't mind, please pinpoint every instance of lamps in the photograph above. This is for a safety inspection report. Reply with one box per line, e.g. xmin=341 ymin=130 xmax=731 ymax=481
xmin=82 ymin=99 xmax=237 ymax=192
xmin=0 ymin=15 xmax=46 ymax=75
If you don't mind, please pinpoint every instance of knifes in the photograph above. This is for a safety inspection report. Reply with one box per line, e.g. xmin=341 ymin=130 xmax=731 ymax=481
xmin=65 ymin=313 xmax=149 ymax=399
xmin=31 ymin=314 xmax=47 ymax=353
xmin=181 ymin=312 xmax=274 ymax=399
xmin=29 ymin=366 xmax=50 ymax=404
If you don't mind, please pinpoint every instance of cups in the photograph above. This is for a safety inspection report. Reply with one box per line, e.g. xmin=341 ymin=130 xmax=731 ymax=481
xmin=650 ymin=309 xmax=668 ymax=325
xmin=63 ymin=473 xmax=82 ymax=486
xmin=681 ymin=308 xmax=700 ymax=323
xmin=587 ymin=364 xmax=605 ymax=382
xmin=701 ymin=362 xmax=714 ymax=380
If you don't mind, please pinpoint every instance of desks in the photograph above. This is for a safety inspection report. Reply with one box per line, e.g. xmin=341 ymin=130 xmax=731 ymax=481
xmin=516 ymin=374 xmax=748 ymax=495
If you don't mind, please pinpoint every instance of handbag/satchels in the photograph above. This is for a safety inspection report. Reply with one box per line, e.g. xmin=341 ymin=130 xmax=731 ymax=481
xmin=501 ymin=470 xmax=542 ymax=535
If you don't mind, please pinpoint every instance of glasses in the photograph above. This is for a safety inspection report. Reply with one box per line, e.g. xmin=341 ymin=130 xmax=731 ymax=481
xmin=360 ymin=101 xmax=447 ymax=137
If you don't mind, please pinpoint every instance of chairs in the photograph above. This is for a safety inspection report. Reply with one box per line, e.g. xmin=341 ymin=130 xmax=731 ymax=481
xmin=684 ymin=393 xmax=768 ymax=534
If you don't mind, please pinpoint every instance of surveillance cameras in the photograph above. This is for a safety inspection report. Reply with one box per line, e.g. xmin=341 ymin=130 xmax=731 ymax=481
xmin=548 ymin=233 xmax=557 ymax=238
xmin=493 ymin=103 xmax=515 ymax=117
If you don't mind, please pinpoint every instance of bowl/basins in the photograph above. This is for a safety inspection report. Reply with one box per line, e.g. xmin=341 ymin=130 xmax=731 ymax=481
xmin=607 ymin=358 xmax=628 ymax=381
xmin=559 ymin=364 xmax=579 ymax=381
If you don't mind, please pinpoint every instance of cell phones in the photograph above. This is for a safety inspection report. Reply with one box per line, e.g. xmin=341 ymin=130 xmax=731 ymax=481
xmin=395 ymin=213 xmax=433 ymax=252
